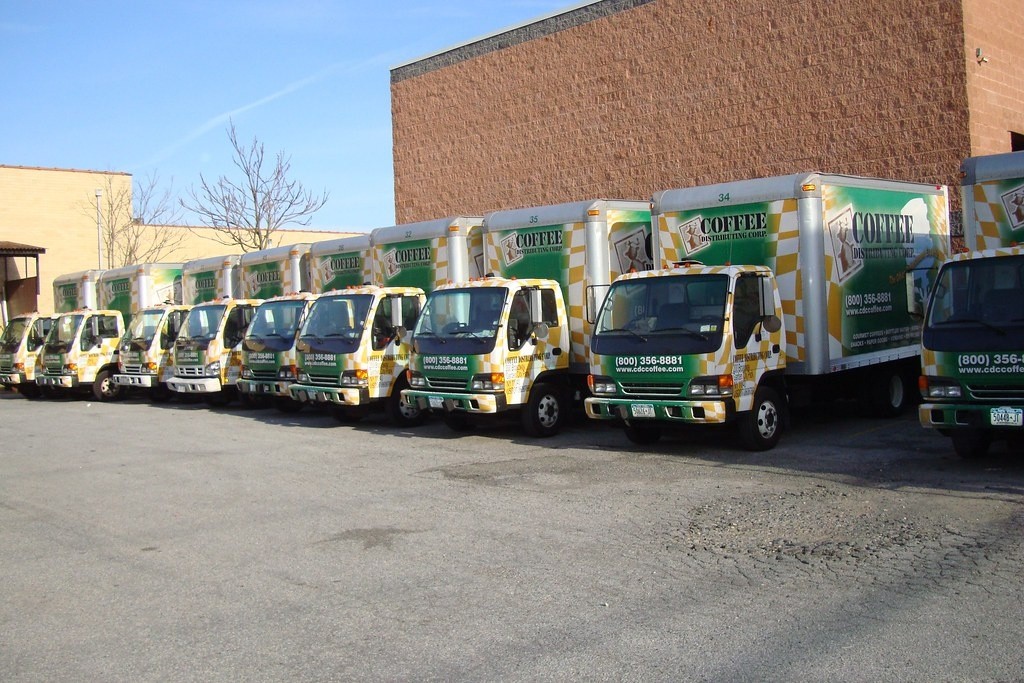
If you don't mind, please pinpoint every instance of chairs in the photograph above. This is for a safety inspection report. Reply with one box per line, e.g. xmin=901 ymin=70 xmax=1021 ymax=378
xmin=967 ymin=280 xmax=1005 ymax=322
xmin=656 ymin=304 xmax=690 ymax=329
xmin=509 ymin=318 xmax=518 ymax=337
xmin=375 ymin=314 xmax=392 ymax=336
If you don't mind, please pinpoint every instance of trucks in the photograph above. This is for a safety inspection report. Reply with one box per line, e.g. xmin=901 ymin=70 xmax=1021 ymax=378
xmin=585 ymin=170 xmax=953 ymax=453
xmin=0 ymin=218 xmax=373 ymax=410
xmin=403 ymin=199 xmax=652 ymax=439
xmin=915 ymin=150 xmax=1024 ymax=459
xmin=287 ymin=214 xmax=486 ymax=428
